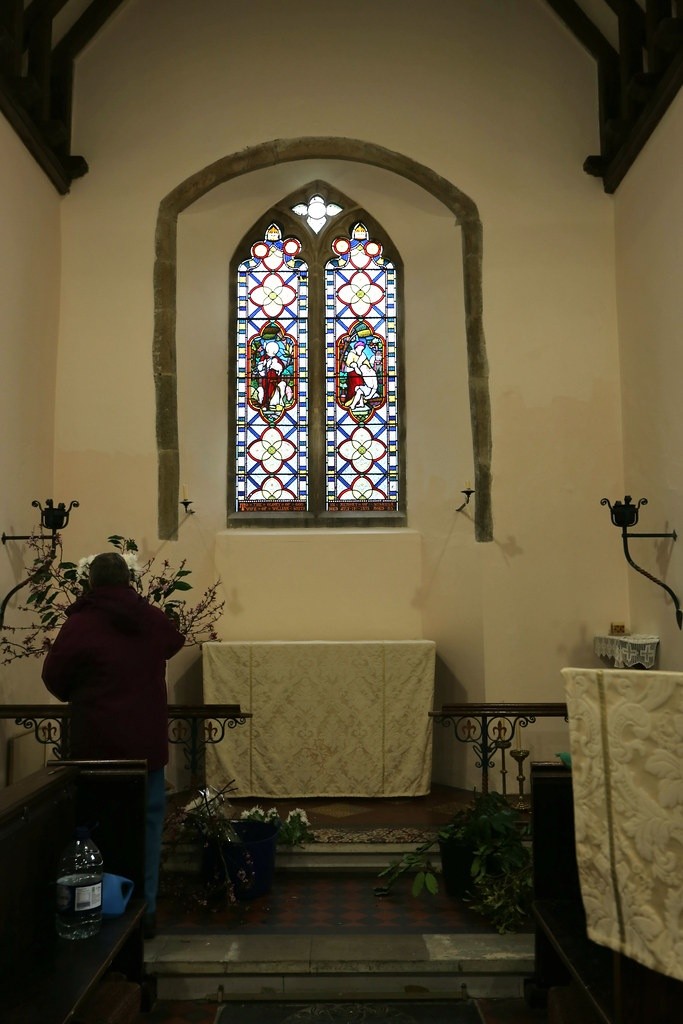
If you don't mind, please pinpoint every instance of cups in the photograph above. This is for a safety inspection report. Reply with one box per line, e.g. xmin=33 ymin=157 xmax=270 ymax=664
xmin=101 ymin=872 xmax=134 ymax=917
xmin=611 ymin=621 xmax=625 ymax=634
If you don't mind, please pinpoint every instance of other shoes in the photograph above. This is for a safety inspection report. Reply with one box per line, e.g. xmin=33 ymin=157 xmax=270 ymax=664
xmin=142 ymin=912 xmax=156 ymax=929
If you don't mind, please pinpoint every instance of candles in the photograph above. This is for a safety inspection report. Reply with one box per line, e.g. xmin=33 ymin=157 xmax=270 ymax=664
xmin=183 ymin=482 xmax=188 ymax=500
xmin=465 ymin=479 xmax=471 ymax=488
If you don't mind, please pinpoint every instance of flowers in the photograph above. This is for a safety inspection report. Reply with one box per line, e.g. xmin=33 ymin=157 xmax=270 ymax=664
xmin=0 ymin=524 xmax=231 ymax=668
xmin=157 ymin=787 xmax=256 ymax=912
xmin=237 ymin=804 xmax=315 ymax=850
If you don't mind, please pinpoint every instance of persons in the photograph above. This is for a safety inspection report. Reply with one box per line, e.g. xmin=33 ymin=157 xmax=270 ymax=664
xmin=42 ymin=552 xmax=187 ymax=942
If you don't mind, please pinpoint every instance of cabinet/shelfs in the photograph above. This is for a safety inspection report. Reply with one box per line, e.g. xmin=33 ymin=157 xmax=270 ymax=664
xmin=524 ymin=760 xmax=683 ymax=1024
xmin=0 ymin=767 xmax=150 ymax=1024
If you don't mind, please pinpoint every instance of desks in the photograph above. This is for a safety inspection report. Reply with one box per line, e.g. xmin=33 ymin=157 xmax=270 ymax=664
xmin=555 ymin=665 xmax=683 ymax=987
xmin=202 ymin=639 xmax=438 ymax=799
xmin=593 ymin=634 xmax=660 ymax=670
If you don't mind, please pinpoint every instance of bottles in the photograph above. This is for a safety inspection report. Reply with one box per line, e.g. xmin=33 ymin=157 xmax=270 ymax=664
xmin=56 ymin=826 xmax=104 ymax=939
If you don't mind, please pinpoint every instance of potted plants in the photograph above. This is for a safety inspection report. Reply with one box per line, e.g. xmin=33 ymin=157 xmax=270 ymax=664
xmin=371 ymin=788 xmax=535 ymax=936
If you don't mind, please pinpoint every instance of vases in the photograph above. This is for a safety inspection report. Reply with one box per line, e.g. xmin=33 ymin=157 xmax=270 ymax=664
xmin=206 ymin=819 xmax=278 ymax=901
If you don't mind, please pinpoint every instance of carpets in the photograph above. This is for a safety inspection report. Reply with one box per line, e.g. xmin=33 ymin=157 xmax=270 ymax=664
xmin=211 ymin=998 xmax=488 ymax=1024
